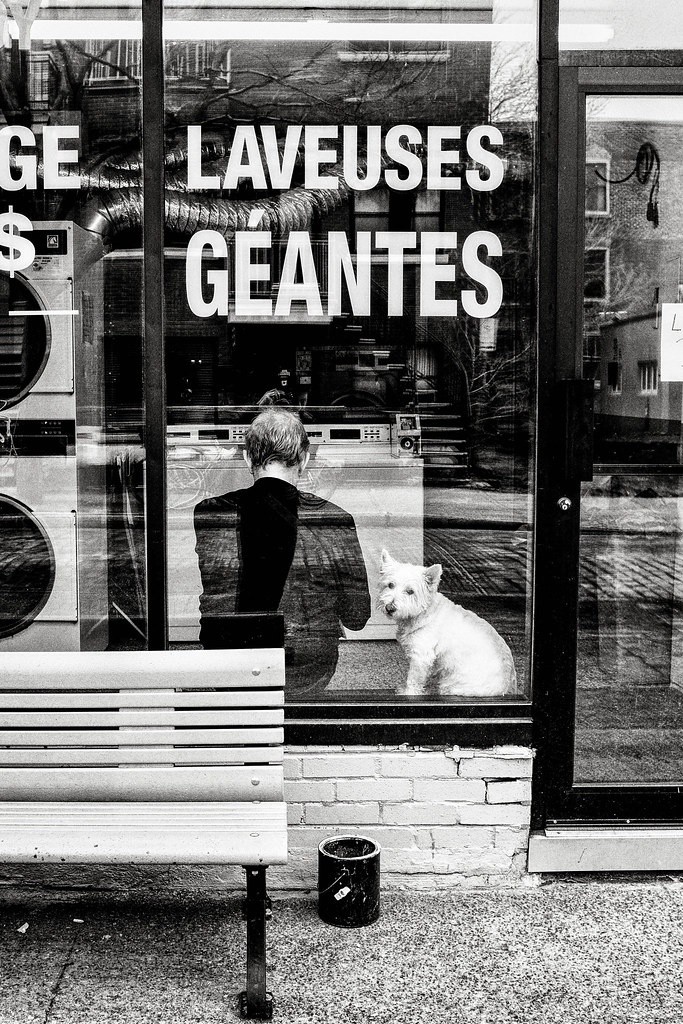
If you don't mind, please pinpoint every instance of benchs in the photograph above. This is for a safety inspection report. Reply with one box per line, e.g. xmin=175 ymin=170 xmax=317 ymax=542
xmin=0 ymin=648 xmax=287 ymax=1021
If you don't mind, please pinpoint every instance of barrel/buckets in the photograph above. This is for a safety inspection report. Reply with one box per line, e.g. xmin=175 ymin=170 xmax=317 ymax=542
xmin=316 ymin=835 xmax=380 ymax=928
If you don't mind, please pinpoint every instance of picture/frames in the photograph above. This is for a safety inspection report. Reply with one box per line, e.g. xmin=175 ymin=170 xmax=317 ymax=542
xmin=585 ymin=246 xmax=610 ymax=303
xmin=585 ymin=159 xmax=611 ymax=216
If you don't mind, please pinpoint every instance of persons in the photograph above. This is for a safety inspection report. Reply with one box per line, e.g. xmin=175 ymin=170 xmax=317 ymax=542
xmin=194 ymin=410 xmax=371 ymax=694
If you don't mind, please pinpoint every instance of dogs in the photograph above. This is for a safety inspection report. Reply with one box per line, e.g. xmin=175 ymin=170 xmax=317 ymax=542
xmin=377 ymin=551 xmax=518 ymax=699
